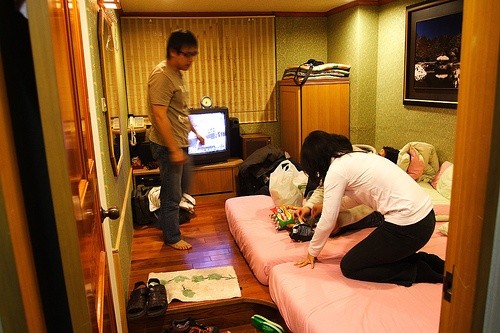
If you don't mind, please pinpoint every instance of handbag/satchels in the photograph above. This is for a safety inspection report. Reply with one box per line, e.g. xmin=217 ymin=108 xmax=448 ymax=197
xmin=307 ymin=58 xmax=325 ymax=66
xmin=135 ymin=185 xmax=156 ymax=226
xmin=269 ymin=160 xmax=308 ymax=208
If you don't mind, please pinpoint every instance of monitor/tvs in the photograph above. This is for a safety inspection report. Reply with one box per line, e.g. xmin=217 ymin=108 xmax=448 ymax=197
xmin=185 ymin=106 xmax=231 ymax=166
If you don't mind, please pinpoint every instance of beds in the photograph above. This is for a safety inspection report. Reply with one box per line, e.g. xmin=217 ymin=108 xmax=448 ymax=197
xmin=224 ymin=161 xmax=453 ymax=286
xmin=268 ymin=231 xmax=447 ymax=331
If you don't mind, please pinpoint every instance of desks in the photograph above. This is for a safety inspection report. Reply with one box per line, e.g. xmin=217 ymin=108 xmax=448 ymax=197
xmin=186 ymin=159 xmax=243 ymax=196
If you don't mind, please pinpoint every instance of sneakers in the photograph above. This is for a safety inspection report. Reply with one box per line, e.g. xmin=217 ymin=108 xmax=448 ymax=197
xmin=168 ymin=318 xmax=219 ymax=333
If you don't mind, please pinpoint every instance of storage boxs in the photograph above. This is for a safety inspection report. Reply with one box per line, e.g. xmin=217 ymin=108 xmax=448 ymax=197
xmin=127 ymin=297 xmax=289 ymax=333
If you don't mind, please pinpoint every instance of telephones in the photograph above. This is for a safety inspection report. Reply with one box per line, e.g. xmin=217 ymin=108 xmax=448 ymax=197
xmin=127 ymin=116 xmax=144 ymax=128
xmin=111 ymin=117 xmax=120 ymax=130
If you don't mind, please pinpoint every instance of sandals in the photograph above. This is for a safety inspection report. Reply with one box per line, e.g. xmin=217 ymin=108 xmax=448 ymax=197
xmin=126 ymin=281 xmax=149 ymax=320
xmin=147 ymin=278 xmax=169 ymax=317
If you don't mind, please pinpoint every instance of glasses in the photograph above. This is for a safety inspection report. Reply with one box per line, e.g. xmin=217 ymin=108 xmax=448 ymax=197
xmin=180 ymin=49 xmax=199 ymax=58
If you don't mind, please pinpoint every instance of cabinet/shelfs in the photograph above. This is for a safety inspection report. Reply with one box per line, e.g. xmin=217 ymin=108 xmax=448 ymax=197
xmin=278 ymin=77 xmax=349 ymax=163
xmin=128 ymin=128 xmax=162 ymax=189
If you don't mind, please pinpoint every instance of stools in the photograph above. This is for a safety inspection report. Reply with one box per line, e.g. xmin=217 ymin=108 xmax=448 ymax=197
xmin=240 ymin=133 xmax=272 ymax=161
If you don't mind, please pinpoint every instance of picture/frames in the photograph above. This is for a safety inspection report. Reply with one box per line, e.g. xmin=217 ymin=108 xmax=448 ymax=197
xmin=402 ymin=0 xmax=463 ymax=110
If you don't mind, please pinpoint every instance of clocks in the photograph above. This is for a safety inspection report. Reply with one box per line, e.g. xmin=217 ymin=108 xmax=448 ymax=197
xmin=200 ymin=96 xmax=212 ymax=109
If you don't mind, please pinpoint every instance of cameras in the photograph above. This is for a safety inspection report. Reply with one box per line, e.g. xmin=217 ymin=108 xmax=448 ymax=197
xmin=307 ymin=59 xmax=324 ymax=66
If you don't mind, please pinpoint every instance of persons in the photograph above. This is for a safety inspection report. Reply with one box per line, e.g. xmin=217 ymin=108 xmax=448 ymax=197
xmin=285 ymin=130 xmax=444 ymax=287
xmin=148 ymin=29 xmax=205 ymax=250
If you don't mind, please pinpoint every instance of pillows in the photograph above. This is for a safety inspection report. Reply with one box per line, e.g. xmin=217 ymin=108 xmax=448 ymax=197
xmin=429 ymin=161 xmax=453 ymax=202
xmin=417 ymin=180 xmax=450 ymax=221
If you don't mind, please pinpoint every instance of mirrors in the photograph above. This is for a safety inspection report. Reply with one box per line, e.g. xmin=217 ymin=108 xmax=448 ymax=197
xmin=99 ymin=9 xmax=125 ymax=177
